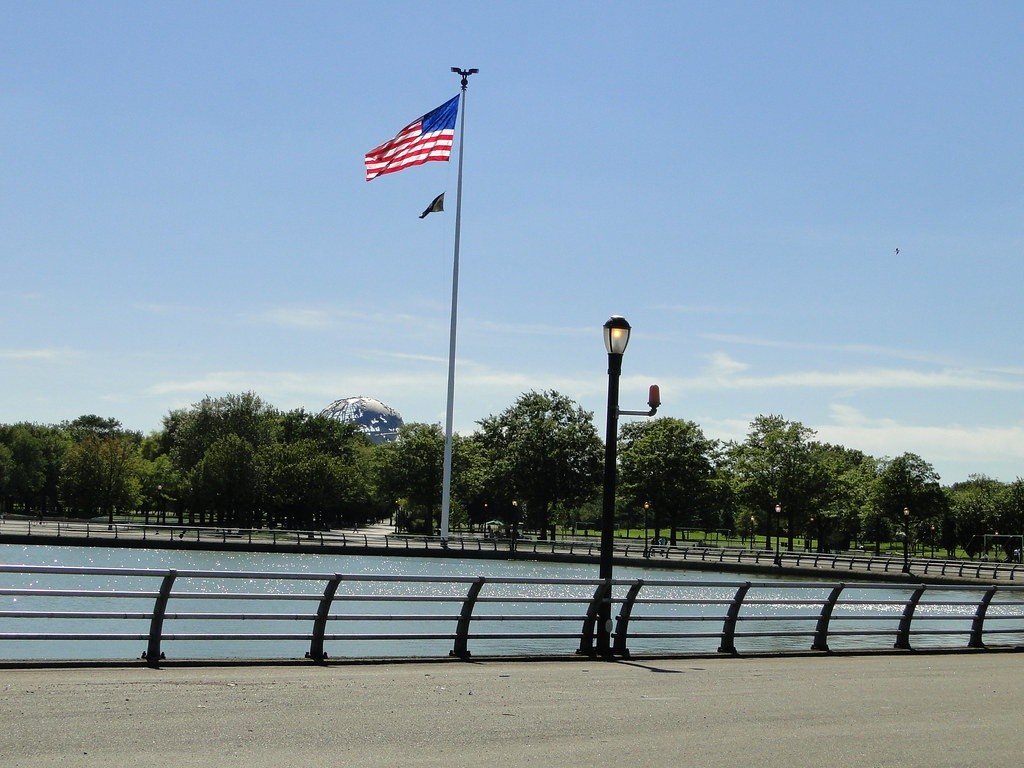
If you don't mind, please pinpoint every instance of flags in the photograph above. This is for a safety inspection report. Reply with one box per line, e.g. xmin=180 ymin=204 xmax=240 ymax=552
xmin=364 ymin=93 xmax=461 ymax=182
xmin=419 ymin=191 xmax=444 ymax=219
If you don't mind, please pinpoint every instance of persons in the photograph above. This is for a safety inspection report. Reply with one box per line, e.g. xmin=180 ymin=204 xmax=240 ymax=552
xmin=36 ymin=511 xmax=43 ymax=525
xmin=2 ymin=510 xmax=7 ymax=524
xmin=649 ymin=536 xmax=668 ymax=556
xmin=981 ymin=547 xmax=1020 ymax=562
xmin=353 ymin=521 xmax=359 ymax=534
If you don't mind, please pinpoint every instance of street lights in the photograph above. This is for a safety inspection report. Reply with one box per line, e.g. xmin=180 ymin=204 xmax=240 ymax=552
xmin=931 ymin=525 xmax=935 ymax=559
xmin=395 ymin=501 xmax=397 ymax=524
xmin=512 ymin=501 xmax=518 ymax=557
xmin=774 ymin=504 xmax=781 ymax=564
xmin=484 ymin=504 xmax=489 ymax=535
xmin=157 ymin=484 xmax=162 ymax=524
xmin=995 ymin=530 xmax=998 ymax=557
xmin=596 ymin=317 xmax=661 ymax=656
xmin=903 ymin=506 xmax=910 ymax=573
xmin=750 ymin=516 xmax=754 ymax=553
xmin=810 ymin=518 xmax=814 ymax=552
xmin=644 ymin=501 xmax=650 ymax=557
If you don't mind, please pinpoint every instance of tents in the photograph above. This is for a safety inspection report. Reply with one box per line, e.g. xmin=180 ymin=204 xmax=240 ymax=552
xmin=483 ymin=520 xmax=524 ymax=535
